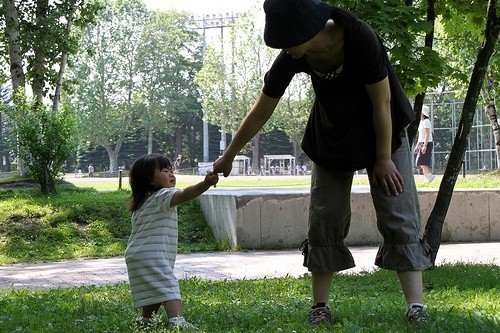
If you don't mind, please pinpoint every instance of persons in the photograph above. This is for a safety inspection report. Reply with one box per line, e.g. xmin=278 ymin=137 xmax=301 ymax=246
xmin=124 ymin=154 xmax=219 ymax=328
xmin=88 ymin=164 xmax=95 ymax=178
xmin=174 ymin=154 xmax=183 ymax=173
xmin=213 ymin=1 xmax=430 ymax=325
xmin=414 ymin=105 xmax=434 ymax=183
xmin=260 ymin=162 xmax=308 ymax=176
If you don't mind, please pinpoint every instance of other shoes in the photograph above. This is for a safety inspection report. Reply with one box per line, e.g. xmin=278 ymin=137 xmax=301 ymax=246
xmin=406 ymin=304 xmax=429 ymax=325
xmin=423 ymin=174 xmax=435 ymax=184
xmin=308 ymin=302 xmax=332 ymax=324
xmin=168 ymin=321 xmax=199 ymax=330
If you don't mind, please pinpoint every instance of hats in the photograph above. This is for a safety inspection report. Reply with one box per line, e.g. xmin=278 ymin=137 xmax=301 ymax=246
xmin=422 ymin=104 xmax=430 ymax=118
xmin=263 ymin=0 xmax=329 ymax=49
